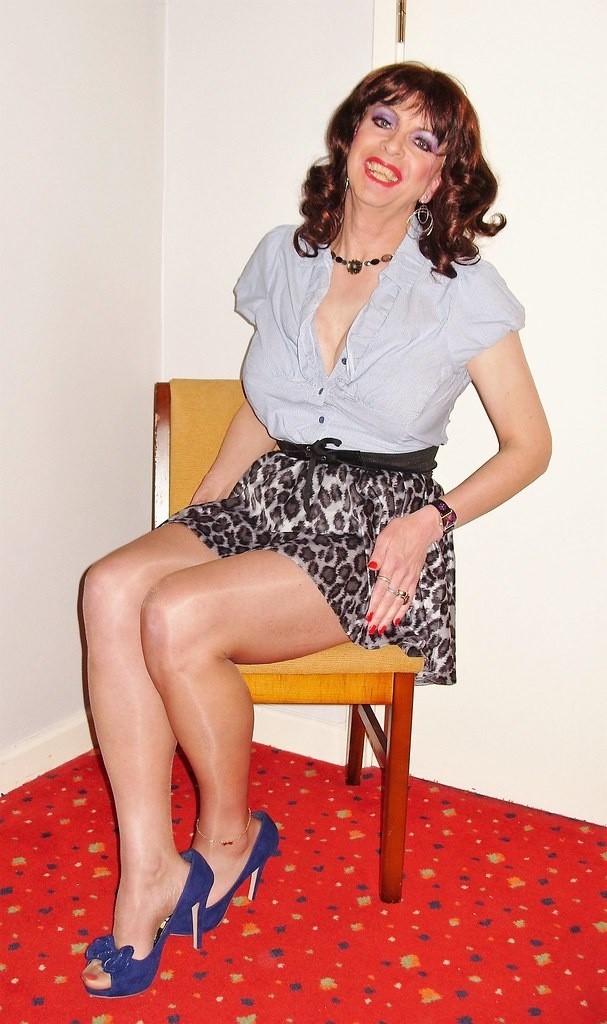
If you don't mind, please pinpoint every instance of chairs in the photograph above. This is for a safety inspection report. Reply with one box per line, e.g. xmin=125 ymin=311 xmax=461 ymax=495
xmin=154 ymin=378 xmax=425 ymax=904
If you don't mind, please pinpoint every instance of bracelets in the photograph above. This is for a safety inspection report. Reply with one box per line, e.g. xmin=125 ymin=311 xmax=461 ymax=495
xmin=428 ymin=498 xmax=457 ymax=536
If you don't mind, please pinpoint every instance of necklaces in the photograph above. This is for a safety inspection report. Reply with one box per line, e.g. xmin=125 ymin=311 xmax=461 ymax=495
xmin=330 ymin=251 xmax=393 ymax=275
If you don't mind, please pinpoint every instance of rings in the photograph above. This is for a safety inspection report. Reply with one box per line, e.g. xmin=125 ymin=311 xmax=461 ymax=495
xmin=387 ymin=587 xmax=397 ymax=596
xmin=396 ymin=589 xmax=410 ymax=605
xmin=377 ymin=576 xmax=391 ymax=583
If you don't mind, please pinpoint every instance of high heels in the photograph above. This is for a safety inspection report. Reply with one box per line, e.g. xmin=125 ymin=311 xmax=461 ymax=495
xmin=167 ymin=810 xmax=280 ymax=936
xmin=79 ymin=848 xmax=215 ymax=998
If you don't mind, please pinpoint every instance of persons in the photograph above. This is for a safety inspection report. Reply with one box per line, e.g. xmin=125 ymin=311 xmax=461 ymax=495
xmin=81 ymin=61 xmax=552 ymax=998
